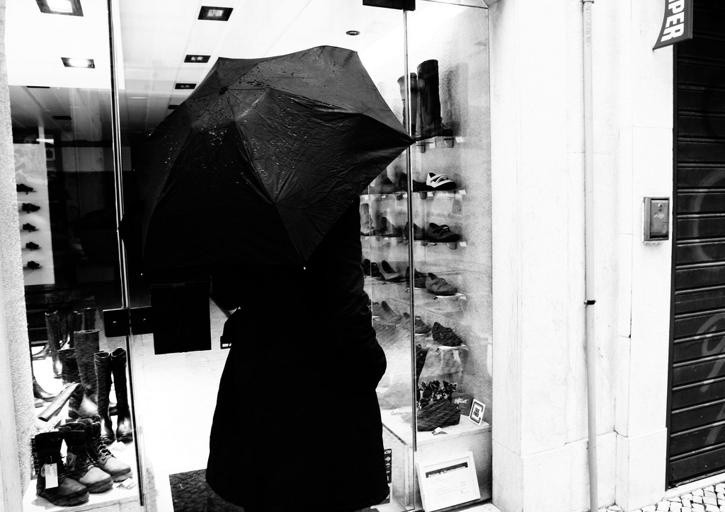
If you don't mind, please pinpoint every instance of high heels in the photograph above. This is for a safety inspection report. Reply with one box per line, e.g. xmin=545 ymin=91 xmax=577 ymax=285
xmin=417 ymin=399 xmax=460 ymax=431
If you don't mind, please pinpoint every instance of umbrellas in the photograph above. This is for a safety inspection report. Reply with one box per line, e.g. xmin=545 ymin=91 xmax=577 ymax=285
xmin=119 ymin=45 xmax=418 ymax=291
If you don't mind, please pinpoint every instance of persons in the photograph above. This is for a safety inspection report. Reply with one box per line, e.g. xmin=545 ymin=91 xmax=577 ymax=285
xmin=205 ymin=197 xmax=390 ymax=512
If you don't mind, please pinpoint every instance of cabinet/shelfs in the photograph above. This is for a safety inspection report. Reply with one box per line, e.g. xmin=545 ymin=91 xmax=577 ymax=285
xmin=363 ymin=138 xmax=468 ymax=512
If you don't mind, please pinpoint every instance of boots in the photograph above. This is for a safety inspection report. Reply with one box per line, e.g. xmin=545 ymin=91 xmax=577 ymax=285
xmin=31 ymin=306 xmax=132 ymax=507
xmin=397 ymin=59 xmax=454 ymax=136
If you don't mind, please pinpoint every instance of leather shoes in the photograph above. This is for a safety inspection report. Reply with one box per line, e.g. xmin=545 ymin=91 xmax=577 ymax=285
xmin=369 ymin=300 xmax=462 ymax=346
xmin=362 ymin=258 xmax=457 ymax=294
xmin=361 ymin=203 xmax=459 ymax=241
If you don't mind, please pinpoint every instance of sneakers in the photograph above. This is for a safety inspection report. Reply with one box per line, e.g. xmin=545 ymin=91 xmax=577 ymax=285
xmin=362 ymin=170 xmax=456 ymax=193
xmin=17 ymin=183 xmax=40 ymax=267
